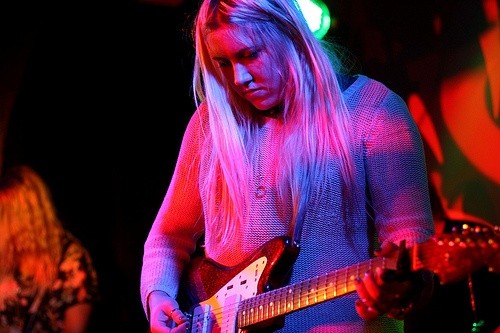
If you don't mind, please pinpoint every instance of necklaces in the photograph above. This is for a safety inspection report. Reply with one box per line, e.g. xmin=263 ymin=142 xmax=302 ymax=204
xmin=256 ymin=120 xmax=275 ymax=198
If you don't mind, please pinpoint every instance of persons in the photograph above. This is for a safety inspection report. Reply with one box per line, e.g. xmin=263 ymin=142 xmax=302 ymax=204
xmin=140 ymin=0 xmax=434 ymax=333
xmin=1 ymin=164 xmax=97 ymax=332
xmin=403 ymin=181 xmax=499 ymax=333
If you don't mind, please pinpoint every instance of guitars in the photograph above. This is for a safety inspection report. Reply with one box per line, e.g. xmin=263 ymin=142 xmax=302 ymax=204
xmin=175 ymin=226 xmax=500 ymax=333
